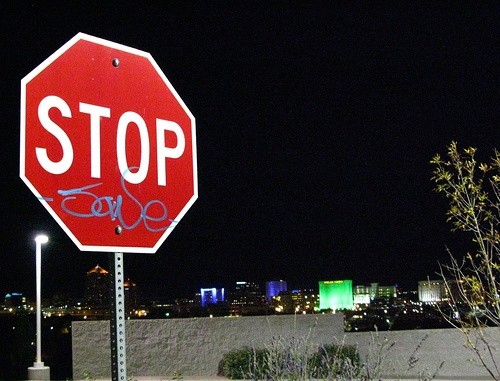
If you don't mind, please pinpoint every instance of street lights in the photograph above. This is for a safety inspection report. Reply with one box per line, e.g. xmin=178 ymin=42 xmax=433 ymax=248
xmin=27 ymin=234 xmax=51 ymax=381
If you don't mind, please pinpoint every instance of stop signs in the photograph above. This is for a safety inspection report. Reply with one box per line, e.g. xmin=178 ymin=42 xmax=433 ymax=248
xmin=21 ymin=32 xmax=199 ymax=253
